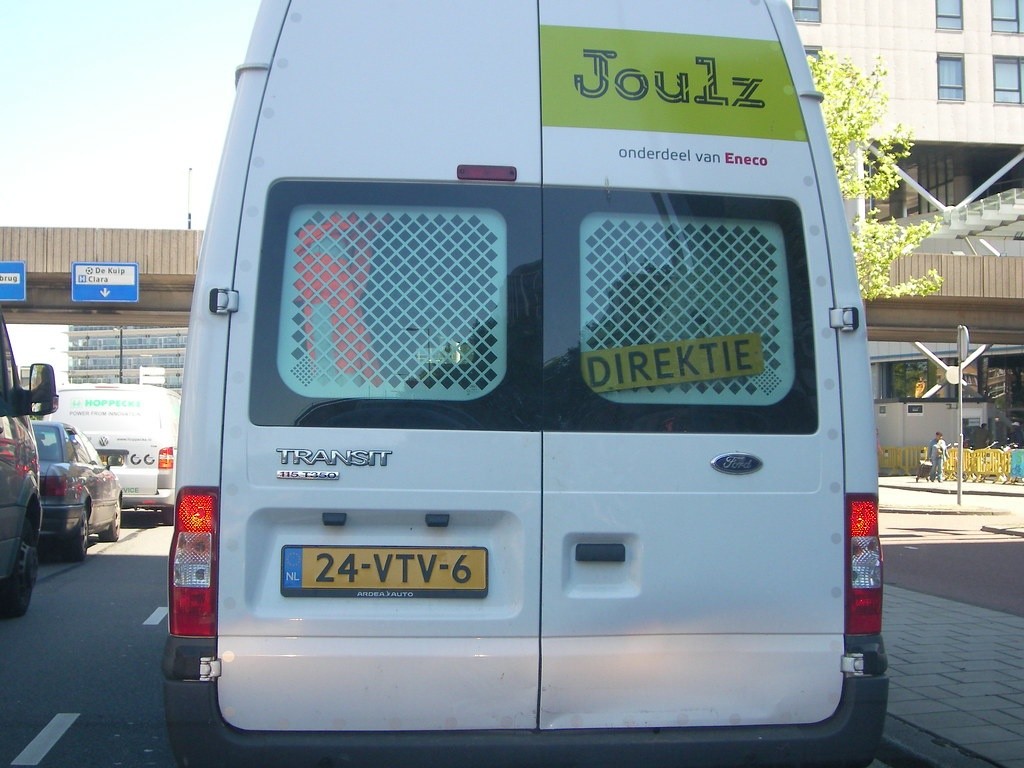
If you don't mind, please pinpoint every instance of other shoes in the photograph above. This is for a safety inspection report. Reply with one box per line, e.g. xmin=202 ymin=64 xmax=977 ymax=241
xmin=931 ymin=480 xmax=934 ymax=482
xmin=938 ymin=479 xmax=943 ymax=483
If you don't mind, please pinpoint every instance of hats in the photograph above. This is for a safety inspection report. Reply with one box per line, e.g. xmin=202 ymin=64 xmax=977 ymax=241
xmin=1012 ymin=421 xmax=1020 ymax=426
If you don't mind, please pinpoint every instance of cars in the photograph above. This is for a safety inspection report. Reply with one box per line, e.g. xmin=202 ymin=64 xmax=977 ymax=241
xmin=30 ymin=418 xmax=123 ymax=561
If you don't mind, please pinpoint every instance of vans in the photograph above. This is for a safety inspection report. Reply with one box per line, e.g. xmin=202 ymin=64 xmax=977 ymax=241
xmin=165 ymin=3 xmax=889 ymax=768
xmin=0 ymin=311 xmax=60 ymax=617
xmin=41 ymin=382 xmax=181 ymax=528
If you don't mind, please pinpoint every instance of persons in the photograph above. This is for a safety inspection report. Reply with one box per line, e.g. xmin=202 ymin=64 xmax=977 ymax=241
xmin=970 ymin=415 xmax=1024 ymax=450
xmin=925 ymin=431 xmax=950 ymax=482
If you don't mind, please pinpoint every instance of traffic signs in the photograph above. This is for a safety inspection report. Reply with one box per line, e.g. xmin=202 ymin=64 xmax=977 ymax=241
xmin=71 ymin=263 xmax=140 ymax=303
